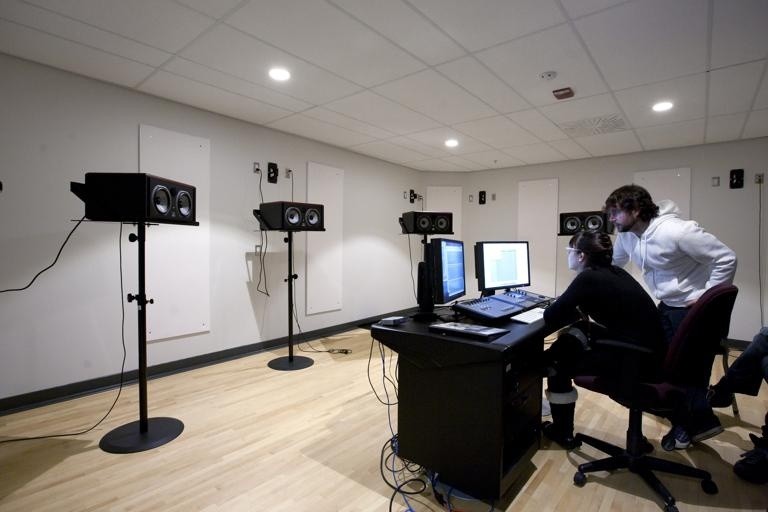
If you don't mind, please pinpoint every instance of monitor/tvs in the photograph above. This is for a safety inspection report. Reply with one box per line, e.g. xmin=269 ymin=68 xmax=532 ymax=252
xmin=424 ymin=238 xmax=466 ymax=304
xmin=474 ymin=241 xmax=530 ymax=296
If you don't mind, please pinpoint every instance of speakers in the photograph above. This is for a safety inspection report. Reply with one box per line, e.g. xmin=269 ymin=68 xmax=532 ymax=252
xmin=399 ymin=211 xmax=452 ymax=234
xmin=412 ymin=262 xmax=440 ymax=324
xmin=253 ymin=201 xmax=324 ymax=229
xmin=71 ymin=173 xmax=196 ymax=222
xmin=560 ymin=211 xmax=614 ymax=233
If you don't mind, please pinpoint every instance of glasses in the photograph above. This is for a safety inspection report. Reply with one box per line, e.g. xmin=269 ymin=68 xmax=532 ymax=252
xmin=565 ymin=247 xmax=578 ymax=254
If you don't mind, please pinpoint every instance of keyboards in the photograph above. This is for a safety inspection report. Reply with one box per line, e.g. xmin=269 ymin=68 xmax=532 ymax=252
xmin=510 ymin=307 xmax=546 ymax=324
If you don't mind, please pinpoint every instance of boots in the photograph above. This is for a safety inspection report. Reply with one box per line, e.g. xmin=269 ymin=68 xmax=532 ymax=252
xmin=542 ymin=327 xmax=587 ymax=379
xmin=542 ymin=387 xmax=578 ymax=450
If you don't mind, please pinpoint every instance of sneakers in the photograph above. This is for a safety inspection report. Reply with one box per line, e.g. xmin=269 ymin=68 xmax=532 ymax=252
xmin=690 ymin=411 xmax=724 ymax=444
xmin=732 ymin=449 xmax=767 ymax=479
xmin=707 ymin=384 xmax=735 ymax=408
xmin=675 ymin=423 xmax=692 ymax=448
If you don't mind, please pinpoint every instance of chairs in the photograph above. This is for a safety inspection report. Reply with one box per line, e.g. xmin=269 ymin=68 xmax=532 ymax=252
xmin=573 ymin=283 xmax=739 ymax=512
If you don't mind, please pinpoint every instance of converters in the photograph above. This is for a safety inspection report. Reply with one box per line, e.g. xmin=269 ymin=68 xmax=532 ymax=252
xmin=381 ymin=316 xmax=408 ymax=327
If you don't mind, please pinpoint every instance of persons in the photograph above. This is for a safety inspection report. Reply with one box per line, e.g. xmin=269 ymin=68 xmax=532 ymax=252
xmin=707 ymin=324 xmax=768 ymax=484
xmin=605 ymin=183 xmax=740 ymax=458
xmin=535 ymin=226 xmax=670 ymax=451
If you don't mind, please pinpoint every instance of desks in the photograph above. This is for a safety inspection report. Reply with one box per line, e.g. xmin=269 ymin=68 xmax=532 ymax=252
xmin=371 ymin=297 xmax=590 ymax=498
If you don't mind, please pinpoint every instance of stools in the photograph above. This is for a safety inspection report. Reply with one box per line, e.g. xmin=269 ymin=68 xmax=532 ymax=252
xmin=715 ymin=337 xmax=738 ymax=415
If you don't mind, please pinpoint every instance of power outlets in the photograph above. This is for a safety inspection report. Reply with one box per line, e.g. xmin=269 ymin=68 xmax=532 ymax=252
xmin=285 ymin=168 xmax=291 ymax=178
xmin=418 ymin=194 xmax=421 ymax=201
xmin=755 ymin=174 xmax=764 ymax=183
xmin=254 ymin=163 xmax=259 ymax=172
